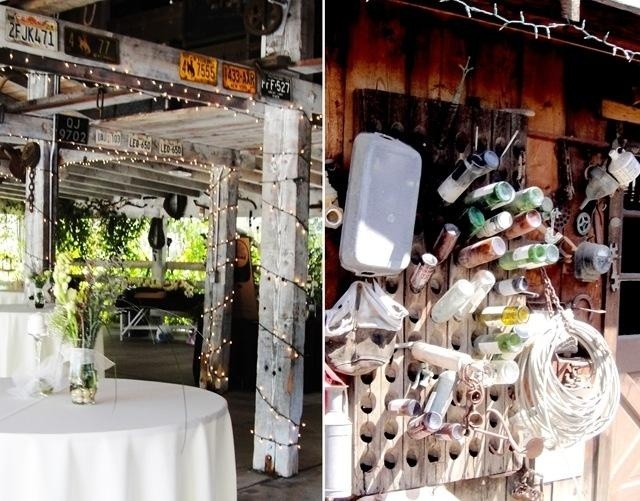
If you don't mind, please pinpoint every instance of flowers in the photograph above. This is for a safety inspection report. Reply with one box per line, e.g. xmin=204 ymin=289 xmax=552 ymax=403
xmin=36 ymin=248 xmax=126 ymax=387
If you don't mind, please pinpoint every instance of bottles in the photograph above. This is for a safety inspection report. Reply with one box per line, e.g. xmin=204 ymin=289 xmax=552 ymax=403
xmin=423 ymin=369 xmax=458 ymax=418
xmin=431 ymin=279 xmax=475 ymax=325
xmin=407 ymin=412 xmax=463 ymax=441
xmin=455 ymin=181 xmax=554 ymax=242
xmin=431 ymin=222 xmax=461 ymax=265
xmin=476 ymin=151 xmax=500 ymax=178
xmin=474 ymin=333 xmax=521 ymax=358
xmin=494 ymin=276 xmax=529 ymax=297
xmin=474 ymin=307 xmax=530 ymax=326
xmin=462 ymin=359 xmax=520 ymax=387
xmin=387 ymin=399 xmax=422 ymax=417
xmin=495 ymin=244 xmax=560 ymax=271
xmin=432 ymin=154 xmax=487 ymax=209
xmin=453 ymin=270 xmax=496 ymax=321
xmin=407 ymin=342 xmax=471 ymax=371
xmin=409 ymin=253 xmax=438 ymax=294
xmin=457 ymin=237 xmax=506 ymax=269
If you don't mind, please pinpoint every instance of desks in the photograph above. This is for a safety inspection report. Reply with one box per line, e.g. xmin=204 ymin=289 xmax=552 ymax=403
xmin=0 ymin=303 xmax=105 ymax=378
xmin=0 ymin=374 xmax=237 ymax=500
xmin=116 ymin=287 xmax=203 ymax=341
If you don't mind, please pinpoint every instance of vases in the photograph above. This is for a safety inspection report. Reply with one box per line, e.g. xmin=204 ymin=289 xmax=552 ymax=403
xmin=68 ymin=338 xmax=99 ymax=404
